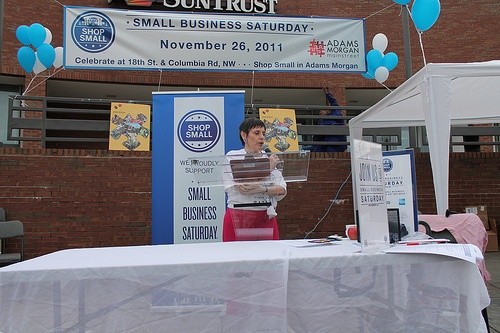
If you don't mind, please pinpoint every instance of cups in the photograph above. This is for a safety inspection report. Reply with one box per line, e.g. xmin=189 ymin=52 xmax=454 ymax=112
xmin=346 ymin=227 xmax=358 ymax=240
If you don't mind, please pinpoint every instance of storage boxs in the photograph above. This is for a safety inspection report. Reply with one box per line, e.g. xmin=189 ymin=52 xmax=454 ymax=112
xmin=464 ymin=205 xmax=499 ymax=253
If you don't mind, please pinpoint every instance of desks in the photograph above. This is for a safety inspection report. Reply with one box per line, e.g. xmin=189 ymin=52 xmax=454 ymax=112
xmin=0 ymin=237 xmax=492 ymax=333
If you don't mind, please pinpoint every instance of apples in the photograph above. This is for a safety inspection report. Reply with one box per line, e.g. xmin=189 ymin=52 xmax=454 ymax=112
xmin=347 ymin=226 xmax=358 ymax=240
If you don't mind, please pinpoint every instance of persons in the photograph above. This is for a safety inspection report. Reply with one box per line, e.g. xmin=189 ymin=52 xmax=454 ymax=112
xmin=310 ymin=80 xmax=344 ymax=152
xmin=222 ymin=117 xmax=287 ymax=242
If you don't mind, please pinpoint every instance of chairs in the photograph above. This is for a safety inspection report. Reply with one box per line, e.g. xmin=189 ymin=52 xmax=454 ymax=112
xmin=0 ymin=207 xmax=25 ymax=267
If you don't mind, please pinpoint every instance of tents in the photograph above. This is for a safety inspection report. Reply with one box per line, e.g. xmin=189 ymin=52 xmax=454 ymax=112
xmin=349 ymin=60 xmax=500 ymax=226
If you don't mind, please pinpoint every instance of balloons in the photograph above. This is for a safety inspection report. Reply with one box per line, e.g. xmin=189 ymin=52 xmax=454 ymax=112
xmin=53 ymin=47 xmax=63 ymax=68
xmin=375 ymin=67 xmax=389 ymax=83
xmin=392 ymin=0 xmax=412 ymax=5
xmin=27 ymin=23 xmax=46 ymax=49
xmin=361 ymin=65 xmax=376 ymax=79
xmin=43 ymin=27 xmax=53 ymax=44
xmin=411 ymin=0 xmax=441 ymax=32
xmin=36 ymin=43 xmax=56 ymax=68
xmin=17 ymin=46 xmax=36 ymax=74
xmin=366 ymin=49 xmax=384 ymax=70
xmin=32 ymin=52 xmax=48 ymax=75
xmin=381 ymin=52 xmax=398 ymax=71
xmin=372 ymin=33 xmax=388 ymax=53
xmin=16 ymin=25 xmax=32 ymax=46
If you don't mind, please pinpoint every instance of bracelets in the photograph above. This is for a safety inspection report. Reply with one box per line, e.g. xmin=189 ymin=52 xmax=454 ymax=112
xmin=263 ymin=187 xmax=268 ymax=195
